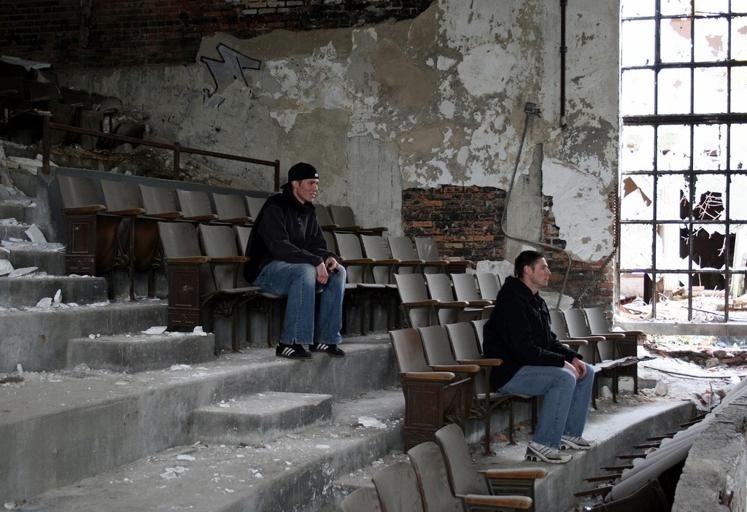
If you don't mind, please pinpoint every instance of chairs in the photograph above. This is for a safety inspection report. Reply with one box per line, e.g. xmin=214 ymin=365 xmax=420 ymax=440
xmin=332 ymin=422 xmax=548 ymax=510
xmin=48 ymin=169 xmax=657 ymax=454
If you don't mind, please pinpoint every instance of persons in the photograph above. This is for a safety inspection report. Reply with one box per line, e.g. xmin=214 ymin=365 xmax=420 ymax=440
xmin=244 ymin=163 xmax=347 ymax=360
xmin=483 ymin=251 xmax=596 ymax=465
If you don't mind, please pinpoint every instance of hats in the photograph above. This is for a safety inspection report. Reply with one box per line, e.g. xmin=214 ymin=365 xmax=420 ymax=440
xmin=279 ymin=163 xmax=318 ymax=188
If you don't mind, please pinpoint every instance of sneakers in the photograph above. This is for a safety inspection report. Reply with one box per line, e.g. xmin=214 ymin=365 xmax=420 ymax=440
xmin=525 ymin=441 xmax=572 ymax=463
xmin=310 ymin=342 xmax=345 ymax=357
xmin=277 ymin=344 xmax=311 ymax=359
xmin=559 ymin=435 xmax=597 ymax=450
